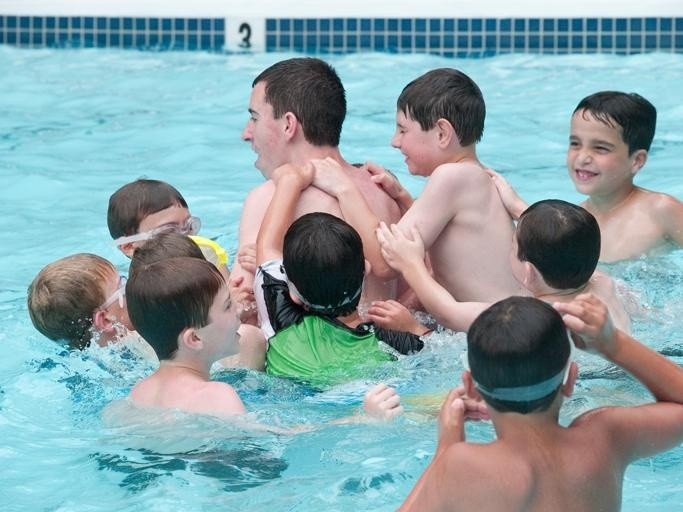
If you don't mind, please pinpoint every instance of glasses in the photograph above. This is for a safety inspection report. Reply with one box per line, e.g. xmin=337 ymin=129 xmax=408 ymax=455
xmin=99 ymin=277 xmax=128 ymax=312
xmin=115 ymin=218 xmax=201 ymax=245
